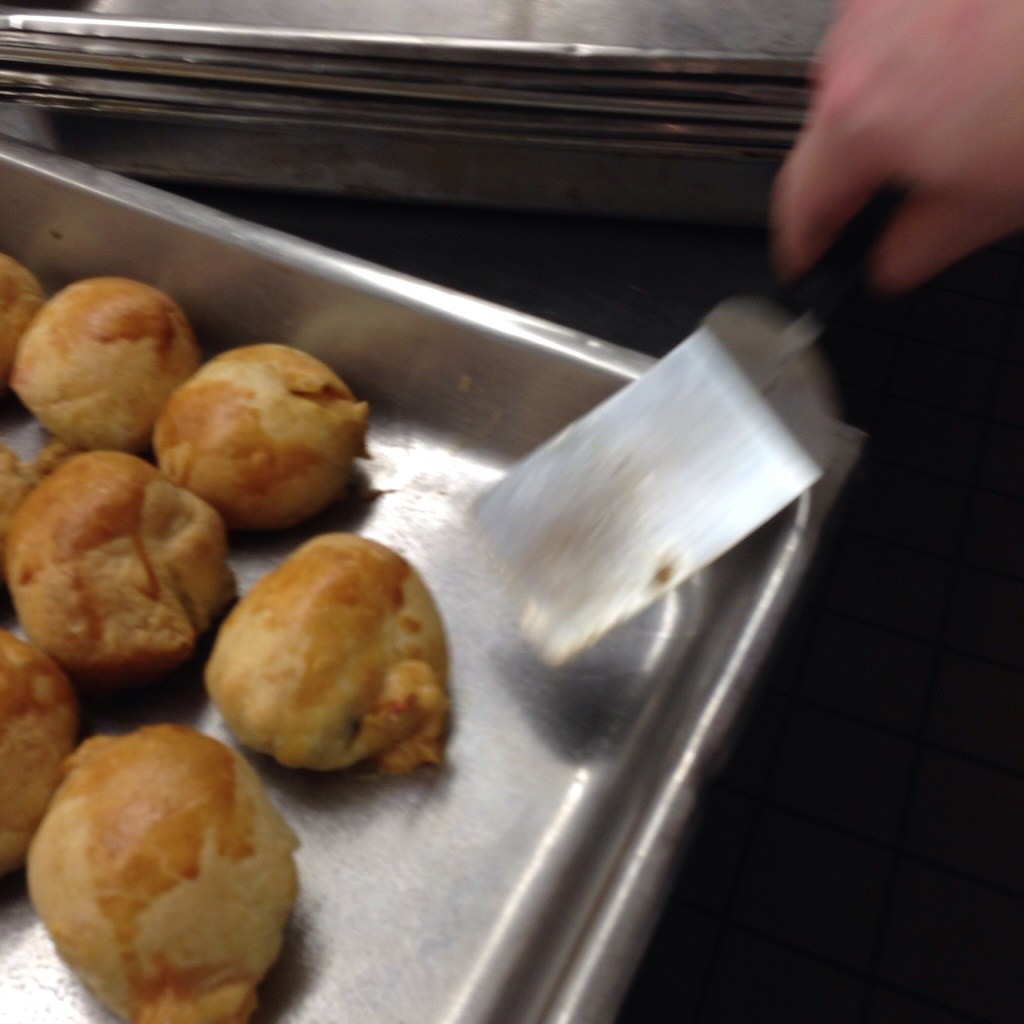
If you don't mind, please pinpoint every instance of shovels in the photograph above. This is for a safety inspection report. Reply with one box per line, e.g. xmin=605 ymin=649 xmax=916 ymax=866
xmin=469 ymin=206 xmax=874 ymax=664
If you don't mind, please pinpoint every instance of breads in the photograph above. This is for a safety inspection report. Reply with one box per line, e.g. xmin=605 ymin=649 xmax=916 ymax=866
xmin=0 ymin=252 xmax=452 ymax=1024
